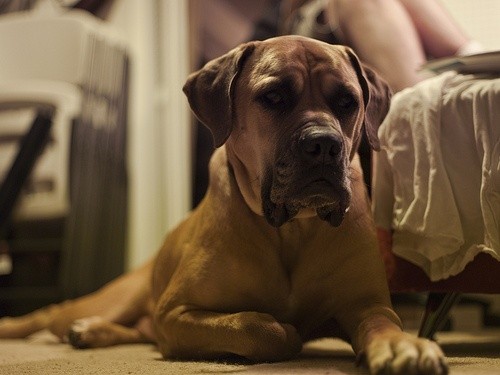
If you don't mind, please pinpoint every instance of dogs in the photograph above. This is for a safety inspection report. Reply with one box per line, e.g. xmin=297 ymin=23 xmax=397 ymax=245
xmin=0 ymin=33 xmax=450 ymax=375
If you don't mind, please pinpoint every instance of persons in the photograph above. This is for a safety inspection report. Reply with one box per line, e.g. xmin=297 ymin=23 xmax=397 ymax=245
xmin=327 ymin=0 xmax=470 ymax=96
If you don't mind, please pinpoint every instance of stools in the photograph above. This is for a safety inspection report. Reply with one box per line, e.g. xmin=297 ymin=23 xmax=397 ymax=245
xmin=372 ymin=71 xmax=500 ymax=341
